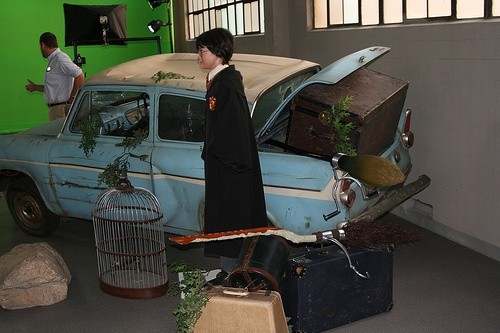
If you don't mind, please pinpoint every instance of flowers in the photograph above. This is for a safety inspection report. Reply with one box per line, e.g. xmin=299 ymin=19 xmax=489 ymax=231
xmin=209 ymin=96 xmax=216 ymax=111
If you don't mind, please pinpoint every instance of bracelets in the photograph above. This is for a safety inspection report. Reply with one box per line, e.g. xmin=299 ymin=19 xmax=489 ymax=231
xmin=70 ymin=95 xmax=75 ymax=98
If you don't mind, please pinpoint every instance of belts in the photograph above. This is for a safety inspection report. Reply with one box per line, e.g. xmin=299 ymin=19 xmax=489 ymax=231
xmin=47 ymin=100 xmax=68 ymax=108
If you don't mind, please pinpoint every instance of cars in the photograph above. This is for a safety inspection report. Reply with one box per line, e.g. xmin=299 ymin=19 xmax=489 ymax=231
xmin=0 ymin=45 xmax=432 ymax=258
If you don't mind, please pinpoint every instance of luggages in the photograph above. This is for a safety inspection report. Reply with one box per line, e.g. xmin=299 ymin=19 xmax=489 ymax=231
xmin=283 ymin=66 xmax=409 ymax=158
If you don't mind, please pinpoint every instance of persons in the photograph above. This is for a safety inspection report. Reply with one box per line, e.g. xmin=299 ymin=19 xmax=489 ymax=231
xmin=197 ymin=27 xmax=268 ymax=287
xmin=25 ymin=32 xmax=85 ymax=122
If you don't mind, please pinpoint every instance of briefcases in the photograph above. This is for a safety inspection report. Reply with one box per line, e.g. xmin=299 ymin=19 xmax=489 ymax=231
xmin=183 ymin=266 xmax=292 ymax=333
xmin=229 ymin=225 xmax=290 ymax=316
xmin=285 ymin=234 xmax=395 ymax=333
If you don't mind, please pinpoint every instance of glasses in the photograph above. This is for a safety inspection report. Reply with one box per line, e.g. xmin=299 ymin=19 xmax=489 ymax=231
xmin=197 ymin=48 xmax=212 ymax=56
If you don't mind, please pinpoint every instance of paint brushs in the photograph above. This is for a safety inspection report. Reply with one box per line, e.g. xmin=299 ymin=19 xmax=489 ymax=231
xmin=266 ymin=139 xmax=405 ymax=187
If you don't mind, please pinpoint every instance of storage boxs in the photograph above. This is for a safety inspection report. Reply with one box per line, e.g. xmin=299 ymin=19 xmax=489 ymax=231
xmin=283 ymin=67 xmax=409 ymax=157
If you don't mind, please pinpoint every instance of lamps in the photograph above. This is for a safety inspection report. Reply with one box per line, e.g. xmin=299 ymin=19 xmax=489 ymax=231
xmin=145 ymin=0 xmax=170 ymax=10
xmin=147 ymin=19 xmax=172 ymax=33
